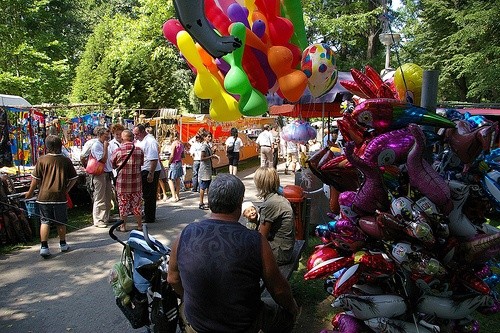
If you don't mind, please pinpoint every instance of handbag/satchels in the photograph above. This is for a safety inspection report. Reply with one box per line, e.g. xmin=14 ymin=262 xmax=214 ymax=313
xmin=86 ymin=154 xmax=104 ymax=176
xmin=150 ymin=257 xmax=179 ymax=333
xmin=227 ymin=146 xmax=234 ymax=152
xmin=108 ymin=248 xmax=150 ymax=329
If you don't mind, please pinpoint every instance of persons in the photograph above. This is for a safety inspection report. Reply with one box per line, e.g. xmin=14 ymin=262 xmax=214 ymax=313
xmin=283 ymin=139 xmax=316 ymax=175
xmin=188 ymin=127 xmax=219 ymax=210
xmin=331 ymin=129 xmax=338 ymax=142
xmin=223 ymin=127 xmax=243 ymax=176
xmin=323 ymin=127 xmax=332 ymax=148
xmin=241 ymin=198 xmax=260 ymax=229
xmin=80 ymin=124 xmax=162 ymax=232
xmin=145 ymin=126 xmax=185 ymax=202
xmin=254 ymin=166 xmax=293 ymax=264
xmin=25 ymin=134 xmax=78 ymax=257
xmin=255 ymin=124 xmax=284 ymax=175
xmin=315 ymin=125 xmax=320 ymax=132
xmin=167 ymin=173 xmax=299 ymax=333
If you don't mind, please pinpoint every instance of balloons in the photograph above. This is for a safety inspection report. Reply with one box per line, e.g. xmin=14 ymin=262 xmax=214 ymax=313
xmin=304 ymin=62 xmax=500 ymax=333
xmin=279 ymin=120 xmax=317 ymax=145
xmin=164 ymin=0 xmax=338 ymax=122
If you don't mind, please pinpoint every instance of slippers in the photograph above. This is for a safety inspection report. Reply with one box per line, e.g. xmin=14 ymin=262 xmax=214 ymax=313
xmin=117 ymin=226 xmax=126 ymax=232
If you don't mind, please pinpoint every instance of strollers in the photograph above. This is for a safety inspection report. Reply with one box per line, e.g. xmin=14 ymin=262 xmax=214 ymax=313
xmin=109 ymin=219 xmax=180 ymax=333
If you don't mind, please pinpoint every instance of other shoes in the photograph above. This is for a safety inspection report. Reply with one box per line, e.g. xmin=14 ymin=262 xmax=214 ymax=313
xmin=141 ymin=220 xmax=153 ymax=223
xmin=167 ymin=197 xmax=179 ymax=202
xmin=40 ymin=248 xmax=50 ymax=256
xmin=61 ymin=244 xmax=69 ymax=251
xmin=95 ymin=224 xmax=107 ymax=228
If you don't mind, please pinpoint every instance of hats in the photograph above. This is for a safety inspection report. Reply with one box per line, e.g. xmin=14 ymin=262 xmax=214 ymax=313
xmin=242 ymin=201 xmax=255 ymax=216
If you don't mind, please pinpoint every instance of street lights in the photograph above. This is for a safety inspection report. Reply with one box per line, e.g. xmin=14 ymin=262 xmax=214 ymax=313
xmin=378 ymin=31 xmax=402 ymax=67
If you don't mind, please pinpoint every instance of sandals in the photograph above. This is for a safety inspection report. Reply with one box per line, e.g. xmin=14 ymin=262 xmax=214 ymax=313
xmin=199 ymin=204 xmax=209 ymax=210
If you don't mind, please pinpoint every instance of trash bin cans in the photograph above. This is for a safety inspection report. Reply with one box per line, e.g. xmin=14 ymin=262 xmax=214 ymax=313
xmin=295 ymin=168 xmax=310 ymax=225
xmin=283 ymin=185 xmax=306 ymax=239
